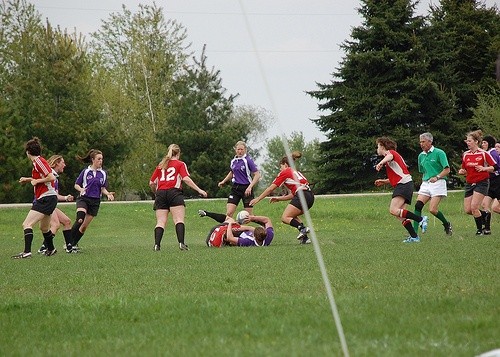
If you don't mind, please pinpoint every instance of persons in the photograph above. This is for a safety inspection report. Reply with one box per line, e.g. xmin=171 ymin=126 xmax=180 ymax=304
xmin=217 ymin=141 xmax=260 ymax=220
xmin=38 ymin=155 xmax=82 ymax=254
xmin=494 ymin=139 xmax=500 ymax=156
xmin=458 ymin=129 xmax=499 ymax=236
xmin=248 ymin=151 xmax=315 ymax=244
xmin=147 ymin=142 xmax=207 ymax=250
xmin=62 ymin=148 xmax=115 ymax=250
xmin=411 ymin=130 xmax=452 ymax=237
xmin=198 ymin=209 xmax=242 ymax=247
xmin=11 ymin=136 xmax=59 ymax=257
xmin=373 ymin=135 xmax=430 ymax=243
xmin=222 ymin=214 xmax=275 ymax=247
xmin=481 ymin=135 xmax=500 ymax=234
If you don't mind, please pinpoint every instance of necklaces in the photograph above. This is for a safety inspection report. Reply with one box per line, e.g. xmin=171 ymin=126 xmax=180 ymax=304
xmin=471 ymin=146 xmax=479 ymax=155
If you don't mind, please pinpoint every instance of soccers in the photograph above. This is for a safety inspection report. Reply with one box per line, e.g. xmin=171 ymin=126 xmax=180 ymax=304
xmin=235 ymin=210 xmax=251 ymax=225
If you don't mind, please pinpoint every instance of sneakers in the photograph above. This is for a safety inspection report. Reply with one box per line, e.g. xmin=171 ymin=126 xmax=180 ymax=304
xmin=9 ymin=251 xmax=33 ymax=259
xmin=296 ymin=227 xmax=312 ymax=244
xmin=38 ymin=243 xmax=57 ymax=257
xmin=475 ymin=229 xmax=492 ymax=236
xmin=179 ymin=242 xmax=193 ymax=251
xmin=442 ymin=221 xmax=453 ymax=236
xmin=62 ymin=243 xmax=84 ymax=255
xmin=153 ymin=245 xmax=164 ymax=252
xmin=402 ymin=235 xmax=421 ymax=243
xmin=419 ymin=215 xmax=429 ymax=235
xmin=198 ymin=208 xmax=207 ymax=217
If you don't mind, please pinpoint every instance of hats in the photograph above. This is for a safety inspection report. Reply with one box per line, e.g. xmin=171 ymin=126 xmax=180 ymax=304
xmin=482 ymin=135 xmax=496 ymax=151
